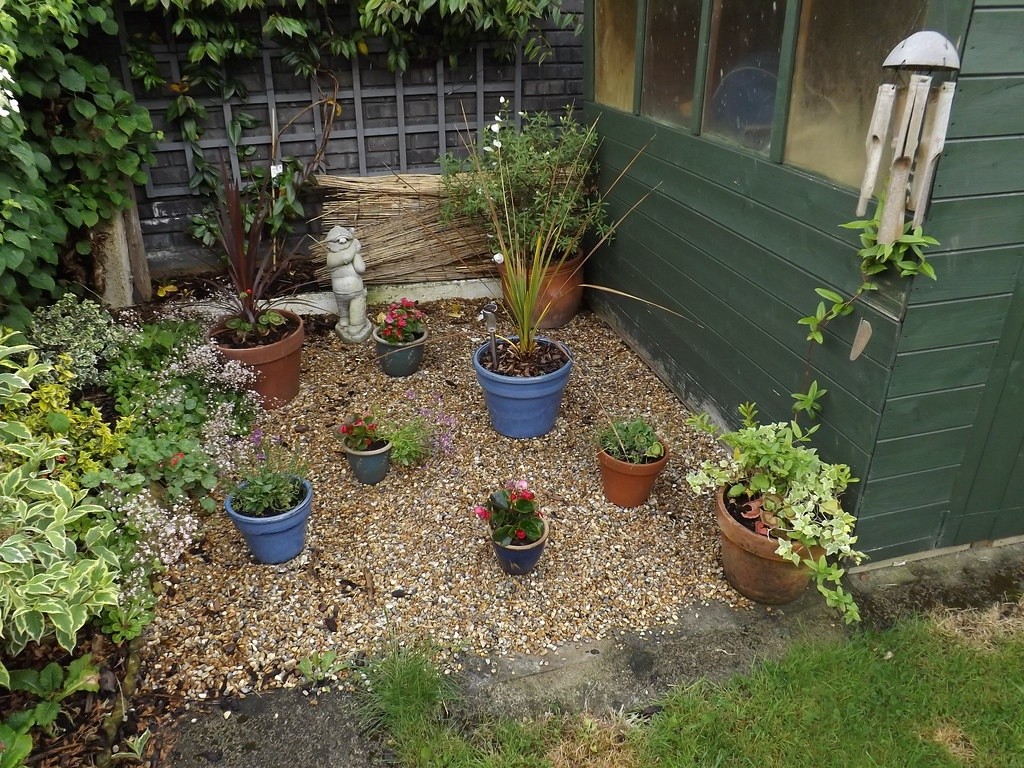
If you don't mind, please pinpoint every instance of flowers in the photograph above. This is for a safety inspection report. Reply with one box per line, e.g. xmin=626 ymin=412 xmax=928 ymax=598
xmin=213 ymin=423 xmax=316 ymax=517
xmin=334 ymin=409 xmax=438 ymax=467
xmin=375 ymin=296 xmax=429 ymax=346
xmin=474 ymin=478 xmax=545 ymax=547
xmin=433 ymin=96 xmax=617 ymax=266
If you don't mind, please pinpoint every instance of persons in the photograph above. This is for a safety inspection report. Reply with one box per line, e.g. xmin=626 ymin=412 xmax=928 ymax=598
xmin=326 ymin=226 xmax=366 ymax=337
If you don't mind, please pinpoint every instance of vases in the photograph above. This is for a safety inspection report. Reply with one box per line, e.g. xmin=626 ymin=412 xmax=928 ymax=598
xmin=342 ymin=436 xmax=394 ymax=485
xmin=487 ymin=515 xmax=549 ymax=575
xmin=493 ymin=248 xmax=585 ymax=327
xmin=224 ymin=473 xmax=313 ymax=566
xmin=372 ymin=327 xmax=428 ymax=377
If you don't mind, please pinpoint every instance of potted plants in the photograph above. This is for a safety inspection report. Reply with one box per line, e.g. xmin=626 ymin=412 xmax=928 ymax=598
xmin=683 ymin=194 xmax=948 ymax=627
xmin=377 ymin=95 xmax=703 ymax=438
xmin=158 ymin=149 xmax=340 ymax=409
xmin=593 ymin=416 xmax=671 ymax=508
